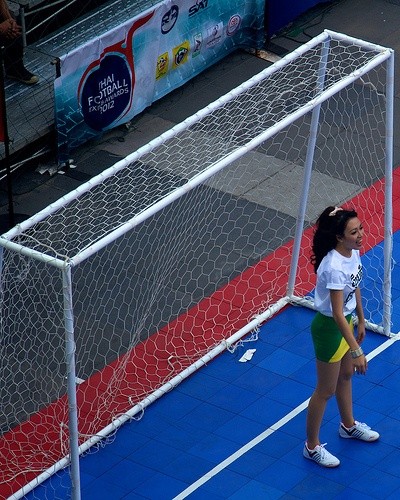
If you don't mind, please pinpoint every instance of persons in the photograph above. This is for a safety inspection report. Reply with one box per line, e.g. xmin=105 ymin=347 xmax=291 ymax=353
xmin=303 ymin=206 xmax=380 ymax=467
xmin=0 ymin=0 xmax=39 ymax=84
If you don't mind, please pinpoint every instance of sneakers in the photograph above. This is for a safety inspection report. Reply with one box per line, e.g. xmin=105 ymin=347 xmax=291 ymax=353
xmin=303 ymin=439 xmax=340 ymax=467
xmin=5 ymin=64 xmax=39 ymax=84
xmin=339 ymin=420 xmax=380 ymax=441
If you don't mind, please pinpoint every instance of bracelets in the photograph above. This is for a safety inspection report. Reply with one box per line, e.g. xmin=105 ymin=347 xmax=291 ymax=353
xmin=350 ymin=347 xmax=363 ymax=359
